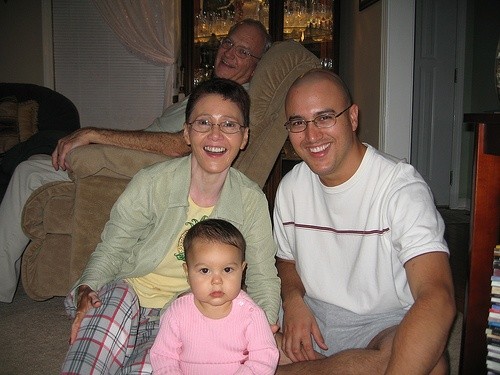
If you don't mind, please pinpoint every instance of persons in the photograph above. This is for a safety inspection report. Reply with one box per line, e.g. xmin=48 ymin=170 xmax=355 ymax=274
xmin=60 ymin=77 xmax=281 ymax=375
xmin=149 ymin=219 xmax=280 ymax=375
xmin=0 ymin=19 xmax=268 ymax=303
xmin=273 ymin=68 xmax=456 ymax=375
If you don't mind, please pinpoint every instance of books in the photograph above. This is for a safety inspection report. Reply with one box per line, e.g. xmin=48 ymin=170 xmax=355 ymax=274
xmin=485 ymin=245 xmax=500 ymax=375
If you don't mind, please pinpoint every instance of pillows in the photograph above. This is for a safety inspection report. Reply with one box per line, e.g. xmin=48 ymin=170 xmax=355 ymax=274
xmin=1 ymin=82 xmax=18 ymax=154
xmin=18 ymin=96 xmax=40 ymax=143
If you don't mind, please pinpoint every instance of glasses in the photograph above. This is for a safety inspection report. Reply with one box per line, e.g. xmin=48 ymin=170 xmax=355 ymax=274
xmin=284 ymin=105 xmax=352 ymax=133
xmin=188 ymin=120 xmax=246 ymax=134
xmin=219 ymin=36 xmax=261 ymax=61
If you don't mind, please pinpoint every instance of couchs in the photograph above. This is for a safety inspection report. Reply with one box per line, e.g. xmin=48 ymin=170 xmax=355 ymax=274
xmin=0 ymin=79 xmax=81 ymax=202
xmin=22 ymin=38 xmax=321 ymax=302
